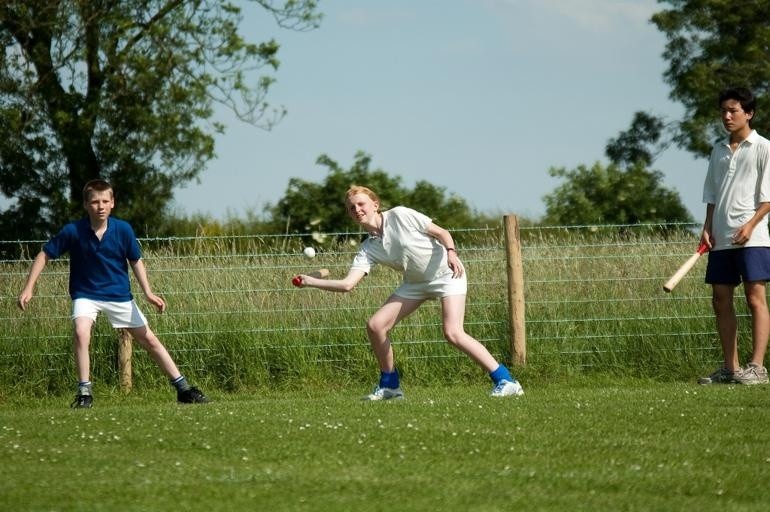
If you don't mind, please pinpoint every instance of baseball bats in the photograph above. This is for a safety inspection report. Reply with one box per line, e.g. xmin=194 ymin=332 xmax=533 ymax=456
xmin=663 ymin=237 xmax=714 ymax=293
xmin=292 ymin=268 xmax=330 ymax=286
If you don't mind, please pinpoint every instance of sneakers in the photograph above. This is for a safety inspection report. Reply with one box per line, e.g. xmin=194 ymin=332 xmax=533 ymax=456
xmin=697 ymin=362 xmax=769 ymax=386
xmin=490 ymin=379 xmax=524 ymax=397
xmin=177 ymin=386 xmax=214 ymax=405
xmin=71 ymin=395 xmax=94 ymax=409
xmin=367 ymin=387 xmax=403 ymax=402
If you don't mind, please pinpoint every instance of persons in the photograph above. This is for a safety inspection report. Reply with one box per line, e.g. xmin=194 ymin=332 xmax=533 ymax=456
xmin=296 ymin=185 xmax=525 ymax=402
xmin=18 ymin=180 xmax=209 ymax=406
xmin=697 ymin=84 xmax=770 ymax=386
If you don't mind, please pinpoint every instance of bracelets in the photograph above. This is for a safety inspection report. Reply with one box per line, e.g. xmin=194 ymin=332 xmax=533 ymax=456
xmin=446 ymin=247 xmax=455 ymax=253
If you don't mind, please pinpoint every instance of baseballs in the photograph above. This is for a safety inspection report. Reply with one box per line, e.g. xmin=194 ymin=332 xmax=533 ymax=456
xmin=304 ymin=246 xmax=316 ymax=260
